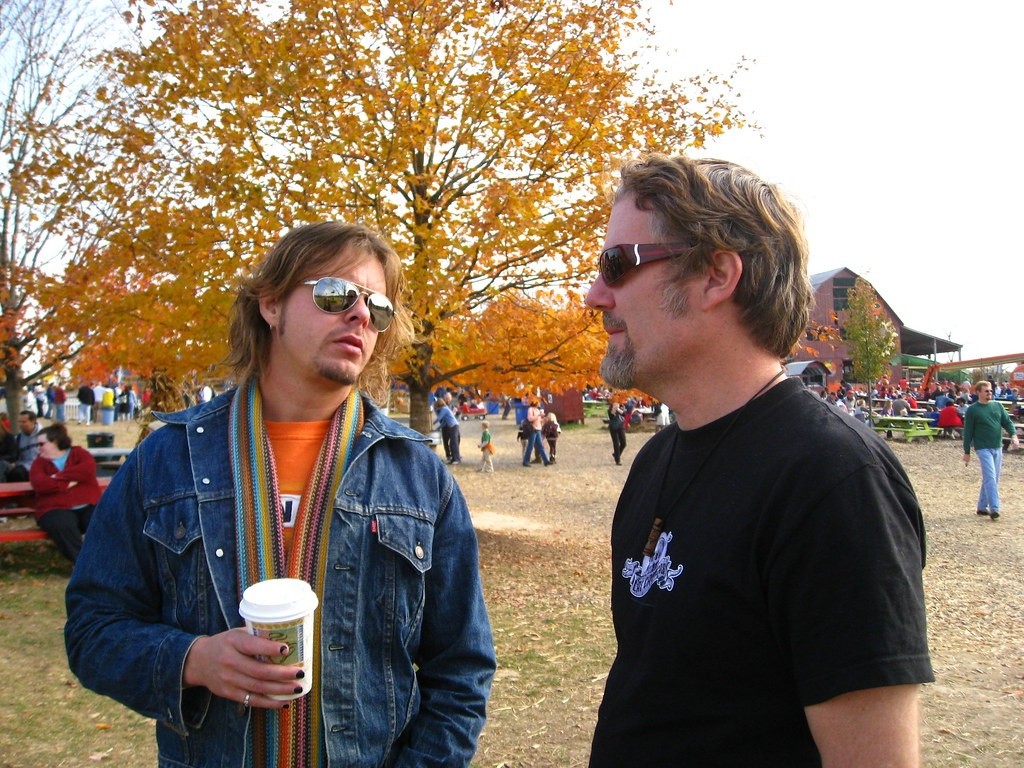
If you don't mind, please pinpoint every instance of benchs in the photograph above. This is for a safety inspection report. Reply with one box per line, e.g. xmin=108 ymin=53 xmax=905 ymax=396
xmin=95 ymin=461 xmax=123 ymax=469
xmin=1 ymin=507 xmax=88 ymax=542
xmin=867 ymin=406 xmax=1024 ymax=452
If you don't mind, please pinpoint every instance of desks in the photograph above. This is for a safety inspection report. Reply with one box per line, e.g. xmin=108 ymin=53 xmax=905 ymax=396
xmin=0 ymin=475 xmax=112 ymax=501
xmin=84 ymin=448 xmax=134 ymax=458
xmin=874 ymin=397 xmax=1024 ymax=452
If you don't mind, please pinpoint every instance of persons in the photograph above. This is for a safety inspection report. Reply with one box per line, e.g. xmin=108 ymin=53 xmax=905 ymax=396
xmin=987 ymin=374 xmax=1011 ymax=397
xmin=517 ymin=398 xmax=561 ymax=467
xmin=23 ymin=375 xmax=218 ymax=426
xmin=819 ymin=379 xmax=876 ymax=427
xmin=431 ymin=392 xmax=461 ymax=465
xmin=870 ymin=374 xmax=918 ymax=417
xmin=608 ymin=401 xmax=627 ymax=465
xmin=342 ymin=289 xmax=357 ymax=310
xmin=923 ymin=376 xmax=977 ymax=409
xmin=963 ymin=380 xmax=1019 ymax=520
xmin=584 ymin=387 xmax=610 ymax=400
xmin=624 ymin=397 xmax=670 ymax=434
xmin=0 ymin=412 xmax=47 ymax=524
xmin=477 ymin=422 xmax=495 ymax=472
xmin=28 ymin=422 xmax=102 ymax=567
xmin=583 ymin=158 xmax=936 ymax=768
xmin=64 ymin=222 xmax=497 ymax=768
xmin=1011 ymin=388 xmax=1018 ymax=409
xmin=451 ymin=387 xmax=512 ymax=420
xmin=938 ymin=398 xmax=969 ymax=439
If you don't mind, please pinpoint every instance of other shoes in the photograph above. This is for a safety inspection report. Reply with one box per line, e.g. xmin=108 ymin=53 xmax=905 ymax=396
xmin=991 ymin=512 xmax=999 ymax=518
xmin=977 ymin=510 xmax=989 ymax=515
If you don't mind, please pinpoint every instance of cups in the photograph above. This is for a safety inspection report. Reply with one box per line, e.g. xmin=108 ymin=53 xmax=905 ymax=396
xmin=239 ymin=578 xmax=319 ymax=700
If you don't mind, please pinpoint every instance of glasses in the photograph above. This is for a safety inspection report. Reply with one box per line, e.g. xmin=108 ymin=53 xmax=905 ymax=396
xmin=597 ymin=242 xmax=695 ymax=286
xmin=299 ymin=276 xmax=397 ymax=331
xmin=980 ymin=390 xmax=993 ymax=392
xmin=39 ymin=440 xmax=51 ymax=446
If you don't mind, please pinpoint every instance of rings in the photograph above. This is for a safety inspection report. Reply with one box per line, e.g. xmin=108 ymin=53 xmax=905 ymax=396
xmin=244 ymin=692 xmax=250 ymax=707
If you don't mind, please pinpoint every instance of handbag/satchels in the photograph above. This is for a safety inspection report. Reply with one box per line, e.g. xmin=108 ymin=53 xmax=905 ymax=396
xmin=611 ymin=415 xmax=625 ymax=430
xmin=522 ymin=420 xmax=531 ymax=439
xmin=542 ymin=421 xmax=558 ymax=437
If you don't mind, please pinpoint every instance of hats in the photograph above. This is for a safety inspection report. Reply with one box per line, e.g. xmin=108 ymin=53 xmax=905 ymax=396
xmin=847 ymin=391 xmax=854 ymax=394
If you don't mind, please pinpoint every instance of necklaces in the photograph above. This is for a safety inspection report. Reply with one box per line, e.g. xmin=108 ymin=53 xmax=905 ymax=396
xmin=641 ymin=370 xmax=787 ymax=556
